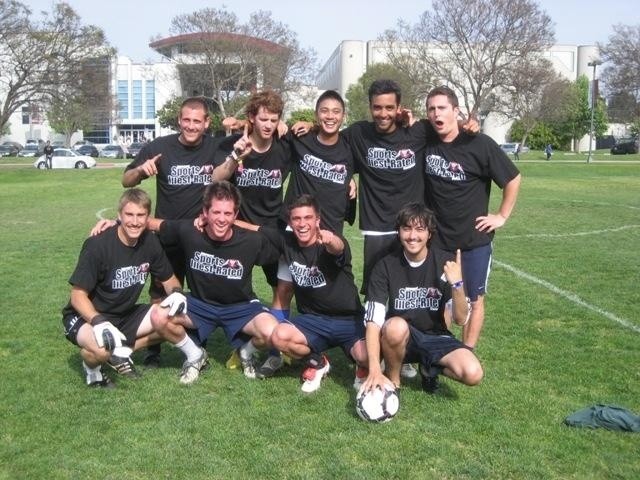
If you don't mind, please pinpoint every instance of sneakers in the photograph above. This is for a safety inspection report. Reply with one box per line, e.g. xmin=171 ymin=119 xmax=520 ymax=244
xmin=400 ymin=362 xmax=417 ymax=379
xmin=143 ymin=352 xmax=162 ymax=371
xmin=83 ymin=361 xmax=112 ymax=389
xmin=180 ymin=347 xmax=207 ymax=386
xmin=107 ymin=348 xmax=138 ymax=379
xmin=354 ymin=365 xmax=370 ymax=391
xmin=300 ymin=355 xmax=332 ymax=393
xmin=237 ymin=346 xmax=256 ymax=379
xmin=419 ymin=363 xmax=438 ymax=393
xmin=257 ymin=355 xmax=284 ymax=379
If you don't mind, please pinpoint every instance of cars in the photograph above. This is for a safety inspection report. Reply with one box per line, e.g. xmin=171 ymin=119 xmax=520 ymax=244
xmin=0 ymin=138 xmax=145 ymax=158
xmin=33 ymin=147 xmax=96 ymax=170
xmin=499 ymin=144 xmax=529 ymax=153
xmin=612 ymin=136 xmax=639 ymax=155
xmin=596 ymin=135 xmax=615 ymax=150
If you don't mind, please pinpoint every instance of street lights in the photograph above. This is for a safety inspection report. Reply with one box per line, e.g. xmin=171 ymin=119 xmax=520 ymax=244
xmin=587 ymin=60 xmax=602 ymax=166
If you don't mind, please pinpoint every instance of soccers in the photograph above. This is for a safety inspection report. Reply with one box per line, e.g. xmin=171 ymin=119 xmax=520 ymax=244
xmin=355 ymin=387 xmax=399 ymax=424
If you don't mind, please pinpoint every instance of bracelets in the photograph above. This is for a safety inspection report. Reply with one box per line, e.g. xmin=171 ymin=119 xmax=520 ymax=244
xmin=228 ymin=151 xmax=241 ymax=162
xmin=451 ymin=280 xmax=464 ymax=289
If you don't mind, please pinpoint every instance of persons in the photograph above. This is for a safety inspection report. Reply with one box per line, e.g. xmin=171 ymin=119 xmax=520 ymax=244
xmin=289 ymin=81 xmax=482 ymax=303
xmin=270 ymin=193 xmax=369 ymax=393
xmin=121 ymin=140 xmax=128 ymax=159
xmin=423 ymin=85 xmax=523 ymax=351
xmin=60 ymin=190 xmax=184 ymax=390
xmin=545 ymin=143 xmax=553 ymax=160
xmin=512 ymin=142 xmax=520 ymax=161
xmin=353 ymin=200 xmax=484 ymax=396
xmin=44 ymin=140 xmax=54 ymax=170
xmin=211 ymin=91 xmax=357 ymax=369
xmin=88 ymin=180 xmax=282 ymax=382
xmin=121 ymin=98 xmax=288 ymax=367
xmin=222 ymin=88 xmax=352 ymax=237
